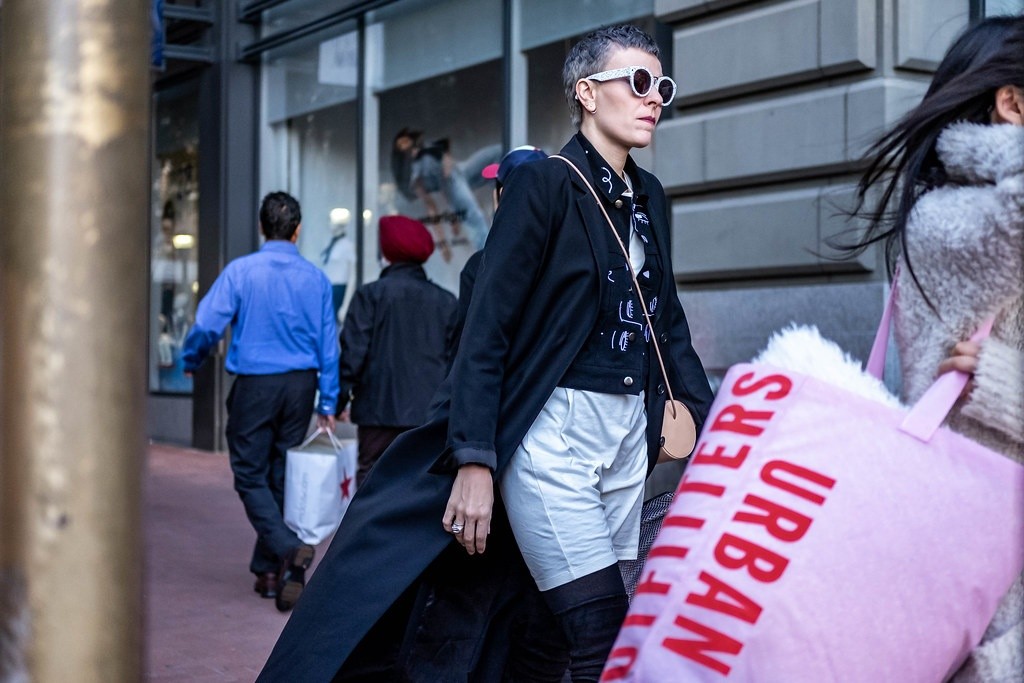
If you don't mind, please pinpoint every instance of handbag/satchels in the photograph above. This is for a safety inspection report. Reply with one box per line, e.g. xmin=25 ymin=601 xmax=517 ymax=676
xmin=600 ymin=258 xmax=1024 ymax=683
xmin=661 ymin=398 xmax=695 ymax=465
xmin=286 ymin=427 xmax=360 ymax=545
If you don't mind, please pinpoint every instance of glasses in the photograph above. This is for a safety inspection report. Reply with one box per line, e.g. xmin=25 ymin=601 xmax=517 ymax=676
xmin=574 ymin=66 xmax=678 ymax=109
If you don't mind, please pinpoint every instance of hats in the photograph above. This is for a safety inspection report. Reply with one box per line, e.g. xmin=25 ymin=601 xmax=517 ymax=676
xmin=379 ymin=213 xmax=435 ymax=265
xmin=481 ymin=144 xmax=549 ymax=184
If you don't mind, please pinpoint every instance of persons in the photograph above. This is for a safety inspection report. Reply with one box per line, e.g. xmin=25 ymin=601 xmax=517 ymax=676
xmin=334 ymin=212 xmax=460 ymax=497
xmin=318 ymin=207 xmax=352 ymax=323
xmin=441 ymin=20 xmax=721 ymax=683
xmin=391 ymin=124 xmax=505 ymax=264
xmin=181 ymin=191 xmax=340 ymax=614
xmin=829 ymin=13 xmax=1024 ymax=683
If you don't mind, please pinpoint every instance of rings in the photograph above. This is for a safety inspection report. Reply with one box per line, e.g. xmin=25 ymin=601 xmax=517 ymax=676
xmin=452 ymin=519 xmax=464 ymax=533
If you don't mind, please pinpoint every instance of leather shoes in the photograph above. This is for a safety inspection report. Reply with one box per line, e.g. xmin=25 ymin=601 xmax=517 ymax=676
xmin=253 ymin=543 xmax=316 ymax=613
